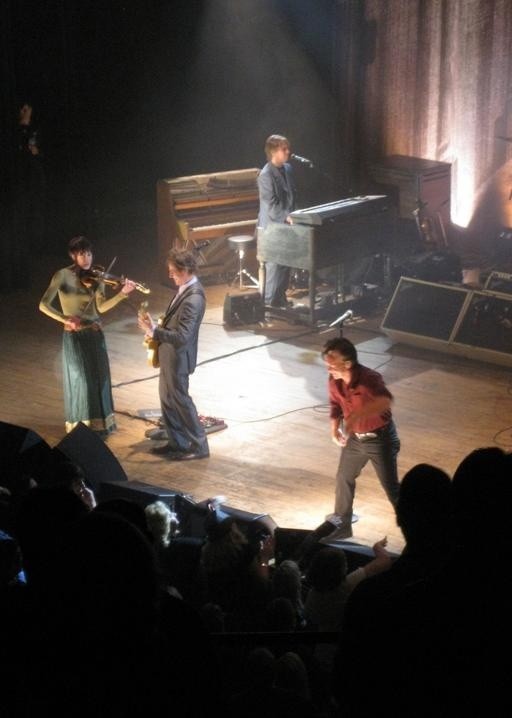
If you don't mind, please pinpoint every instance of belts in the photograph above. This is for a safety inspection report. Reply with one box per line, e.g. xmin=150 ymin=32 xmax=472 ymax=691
xmin=354 ymin=432 xmax=377 ymax=439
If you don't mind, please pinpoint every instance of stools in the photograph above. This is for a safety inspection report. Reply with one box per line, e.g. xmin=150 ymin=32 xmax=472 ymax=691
xmin=226 ymin=235 xmax=259 ymax=291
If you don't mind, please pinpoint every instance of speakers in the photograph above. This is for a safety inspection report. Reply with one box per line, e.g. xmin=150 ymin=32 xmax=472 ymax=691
xmin=224 ymin=292 xmax=265 ymax=325
xmin=102 ymin=478 xmax=398 ymax=575
xmin=382 ymin=275 xmax=512 ymax=368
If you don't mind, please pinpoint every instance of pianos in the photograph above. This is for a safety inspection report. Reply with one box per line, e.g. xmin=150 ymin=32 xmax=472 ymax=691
xmin=287 ymin=194 xmax=397 ymax=226
xmin=156 ymin=168 xmax=262 ymax=291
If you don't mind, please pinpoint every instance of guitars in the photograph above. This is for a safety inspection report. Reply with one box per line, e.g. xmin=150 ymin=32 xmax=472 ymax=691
xmin=140 ymin=301 xmax=165 ymax=368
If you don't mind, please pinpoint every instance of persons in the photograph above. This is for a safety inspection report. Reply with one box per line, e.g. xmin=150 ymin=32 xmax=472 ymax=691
xmin=13 ymin=102 xmax=37 ymax=160
xmin=255 ymin=134 xmax=295 ymax=306
xmin=40 ymin=234 xmax=134 ymax=435
xmin=137 ymin=249 xmax=206 ymax=460
xmin=318 ymin=338 xmax=401 ymax=544
xmin=0 ymin=448 xmax=511 ymax=718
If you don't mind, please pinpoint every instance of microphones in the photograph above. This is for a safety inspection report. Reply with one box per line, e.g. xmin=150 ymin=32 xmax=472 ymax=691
xmin=290 ymin=153 xmax=312 ymax=165
xmin=328 ymin=307 xmax=353 ymax=329
xmin=194 ymin=239 xmax=210 ymax=250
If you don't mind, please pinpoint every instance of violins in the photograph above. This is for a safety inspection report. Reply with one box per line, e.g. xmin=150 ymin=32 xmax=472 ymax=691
xmin=79 ymin=265 xmax=150 ymax=294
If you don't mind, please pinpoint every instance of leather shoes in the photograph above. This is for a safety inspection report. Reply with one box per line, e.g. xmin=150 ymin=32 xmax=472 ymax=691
xmin=172 ymin=450 xmax=198 ymax=461
xmin=321 ymin=522 xmax=352 ymax=542
xmin=152 ymin=445 xmax=175 ymax=454
